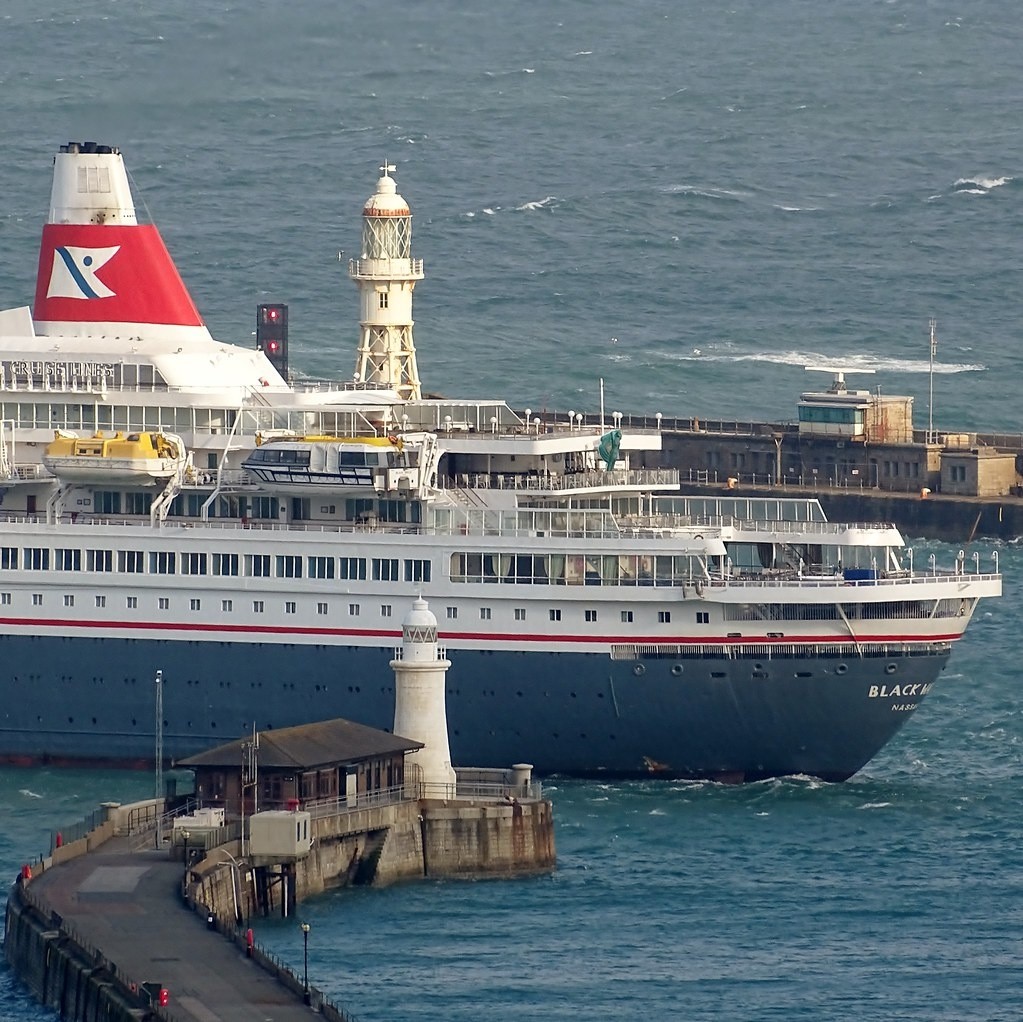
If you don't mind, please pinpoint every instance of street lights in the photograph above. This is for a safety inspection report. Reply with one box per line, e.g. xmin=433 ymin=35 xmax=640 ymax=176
xmin=155 ymin=670 xmax=163 ymax=798
xmin=182 ymin=830 xmax=189 ymax=874
xmin=301 ymin=921 xmax=313 ymax=1006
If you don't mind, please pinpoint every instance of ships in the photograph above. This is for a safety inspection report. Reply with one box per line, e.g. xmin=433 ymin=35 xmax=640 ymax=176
xmin=0 ymin=139 xmax=1004 ymax=785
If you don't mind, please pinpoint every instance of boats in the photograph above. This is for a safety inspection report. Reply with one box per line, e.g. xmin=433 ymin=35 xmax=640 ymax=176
xmin=43 ymin=430 xmax=194 ymax=486
xmin=240 ymin=436 xmax=420 ymax=500
